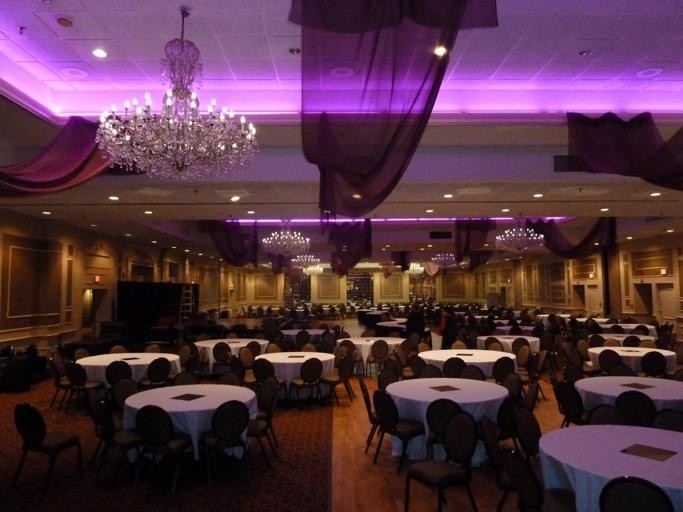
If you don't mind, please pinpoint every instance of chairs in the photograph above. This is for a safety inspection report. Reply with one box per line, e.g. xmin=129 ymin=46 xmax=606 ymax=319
xmin=558 ymin=337 xmax=582 ymax=368
xmin=320 ymin=353 xmax=353 ymax=406
xmin=139 ymin=358 xmax=168 ymax=387
xmin=484 ymin=337 xmax=497 ymax=346
xmin=491 ymin=357 xmax=515 ymax=386
xmin=218 ymin=373 xmax=241 ymax=386
xmin=310 ymin=334 xmax=321 ymax=348
xmin=253 ymin=359 xmax=287 ymax=413
xmin=633 ymin=325 xmax=649 ymax=335
xmin=319 ymin=324 xmax=329 ymax=330
xmin=614 ymin=391 xmax=656 ymax=427
xmin=604 ymin=338 xmax=621 ymax=346
xmin=496 ymin=451 xmax=545 ymax=512
xmin=419 ymin=365 xmax=442 ymax=377
xmin=365 ymin=341 xmax=389 ymax=379
xmin=430 ymin=329 xmax=443 ymax=349
xmin=508 ymin=380 xmax=538 ymax=461
xmin=656 ymin=337 xmax=675 ymax=351
xmin=508 ymin=320 xmax=518 ymax=326
xmin=111 ymin=345 xmax=128 ymax=353
xmin=175 ymin=372 xmax=200 ymax=384
xmin=74 ymin=348 xmax=90 ymax=359
xmin=265 ymin=343 xmax=282 ymax=353
xmin=212 ymin=342 xmax=232 ymax=381
xmin=531 ymin=326 xmax=545 ymax=336
xmin=301 ymin=343 xmax=317 ymax=352
xmin=247 ymin=384 xmax=281 ymax=465
xmin=93 ymin=361 xmax=135 ymax=426
xmin=372 ymin=389 xmax=426 ymax=475
xmin=640 ymin=339 xmax=656 ymax=348
xmin=406 ymin=355 xmax=425 ymax=377
xmin=564 ymin=363 xmax=584 ymax=385
xmin=553 ymin=381 xmax=583 ymax=428
xmin=239 ymin=347 xmax=257 ymax=389
xmin=378 ymin=368 xmax=399 ymax=391
xmin=289 ymin=359 xmax=325 ymax=410
xmin=608 ymin=326 xmax=625 ymax=334
xmin=333 ymin=325 xmax=340 ymax=336
xmin=669 ymin=368 xmax=683 ymax=381
xmin=500 ymin=373 xmax=521 ymax=396
xmin=144 ymin=344 xmax=161 ymax=353
xmin=599 ymin=476 xmax=669 ymax=512
xmin=425 ymin=398 xmax=466 ymax=462
xmin=133 ymin=405 xmax=192 ymax=494
xmin=49 ymin=345 xmax=68 ymax=376
xmin=258 ymin=377 xmax=280 ymax=449
xmin=511 ymin=338 xmax=530 ymax=356
xmin=598 ymin=349 xmax=622 ymax=370
xmin=586 ymin=318 xmax=603 ymax=334
xmin=589 ymin=335 xmax=605 ymax=346
xmin=247 ymin=341 xmax=261 ymax=357
xmin=255 ymin=332 xmax=265 ymax=339
xmin=641 ymin=352 xmax=667 ymax=377
xmin=588 ymin=404 xmax=627 ymax=425
xmin=518 ymin=345 xmax=548 ymax=402
xmin=578 ymin=340 xmax=590 ymax=360
xmin=459 ymin=366 xmax=485 ymax=381
xmin=610 ymin=362 xmax=636 ymax=376
xmin=334 ymin=340 xmax=357 ymax=399
xmin=510 ymin=326 xmax=522 ymax=335
xmin=480 ymin=419 xmax=527 ymax=461
xmin=653 ymin=408 xmax=683 ymax=431
xmin=47 ymin=356 xmax=71 ymax=407
xmin=485 ymin=340 xmax=503 ymax=350
xmin=10 ymin=403 xmax=83 ymax=498
xmin=451 ymin=341 xmax=466 ymax=348
xmin=196 ymin=401 xmax=251 ymax=489
xmin=177 ymin=343 xmax=198 ymax=371
xmin=88 ymin=399 xmax=142 ymax=492
xmin=113 ymin=379 xmax=138 ymax=407
xmin=224 ymin=332 xmax=239 ymax=338
xmin=64 ymin=363 xmax=104 ymax=415
xmin=403 ymin=412 xmax=479 ymax=512
xmin=296 ymin=330 xmax=309 ymax=351
xmin=443 ymin=358 xmax=468 ymax=378
xmin=284 ymin=335 xmax=295 ymax=351
xmin=357 ymin=378 xmax=379 ymax=454
xmin=623 ymin=336 xmax=641 ymax=347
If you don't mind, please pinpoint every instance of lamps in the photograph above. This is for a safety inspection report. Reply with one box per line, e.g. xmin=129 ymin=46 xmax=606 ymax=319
xmin=494 ymin=217 xmax=545 ymax=261
xmin=431 ymin=253 xmax=456 ymax=269
xmin=290 ymin=254 xmax=321 ymax=271
xmin=260 ymin=218 xmax=310 ymax=258
xmin=94 ymin=7 xmax=261 ymax=185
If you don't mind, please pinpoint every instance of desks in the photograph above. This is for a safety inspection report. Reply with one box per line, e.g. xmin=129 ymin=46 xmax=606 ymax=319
xmin=418 ymin=348 xmax=517 ymax=379
xmin=588 ymin=346 xmax=677 ymax=372
xmin=376 ymin=318 xmax=429 ymax=339
xmin=536 ymin=313 xmax=571 ymax=325
xmin=587 ymin=333 xmax=655 ymax=346
xmin=337 ymin=337 xmax=405 ymax=376
xmin=123 ymin=384 xmax=258 ymax=470
xmin=386 ymin=378 xmax=510 ymax=466
xmin=77 ymin=353 xmax=181 ymax=388
xmin=540 ymin=422 xmax=683 ymax=511
xmin=194 ymin=338 xmax=269 ymax=372
xmin=599 ymin=323 xmax=657 ymax=336
xmin=281 ymin=329 xmax=335 ymax=346
xmin=568 ymin=317 xmax=609 ymax=326
xmin=572 ymin=376 xmax=683 ymax=425
xmin=255 ymin=351 xmax=335 ymax=401
xmin=497 ymin=326 xmax=534 ymax=335
xmin=493 ymin=320 xmax=521 ymax=325
xmin=476 ymin=334 xmax=540 ymax=354
